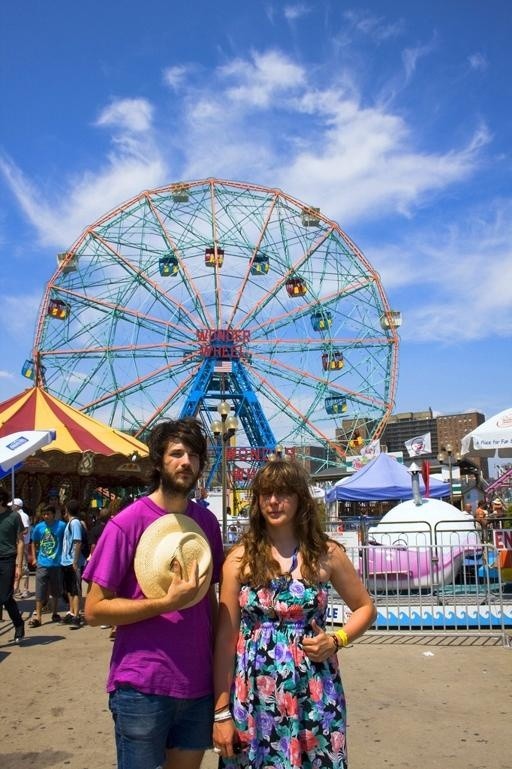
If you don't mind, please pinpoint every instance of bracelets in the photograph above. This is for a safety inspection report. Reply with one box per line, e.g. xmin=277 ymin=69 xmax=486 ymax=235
xmin=327 ymin=628 xmax=350 ymax=653
xmin=212 ymin=703 xmax=234 ymax=723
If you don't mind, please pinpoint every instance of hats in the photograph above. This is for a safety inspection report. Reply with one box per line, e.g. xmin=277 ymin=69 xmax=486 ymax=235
xmin=7 ymin=498 xmax=24 ymax=506
xmin=133 ymin=513 xmax=214 ymax=611
xmin=48 ymin=489 xmax=59 ymax=497
xmin=491 ymin=498 xmax=501 ymax=505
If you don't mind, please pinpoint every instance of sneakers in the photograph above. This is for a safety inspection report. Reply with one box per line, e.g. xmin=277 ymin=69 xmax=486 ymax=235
xmin=14 ymin=621 xmax=24 ymax=637
xmin=52 ymin=611 xmax=82 ymax=629
xmin=13 ymin=590 xmax=33 ymax=598
xmin=28 ymin=619 xmax=41 ymax=628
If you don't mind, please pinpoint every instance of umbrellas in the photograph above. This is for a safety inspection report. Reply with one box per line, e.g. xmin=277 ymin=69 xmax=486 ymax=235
xmin=0 ymin=383 xmax=157 ymax=478
xmin=0 ymin=431 xmax=59 ymax=477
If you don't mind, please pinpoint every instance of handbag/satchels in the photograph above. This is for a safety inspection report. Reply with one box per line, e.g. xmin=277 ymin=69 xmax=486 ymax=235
xmin=68 ymin=517 xmax=90 ymax=558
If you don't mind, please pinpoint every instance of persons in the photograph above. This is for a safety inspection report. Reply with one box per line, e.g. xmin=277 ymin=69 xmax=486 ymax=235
xmin=0 ymin=487 xmax=134 ymax=642
xmin=212 ymin=461 xmax=379 ymax=769
xmin=81 ymin=418 xmax=226 ymax=769
xmin=460 ymin=497 xmax=505 ymax=543
